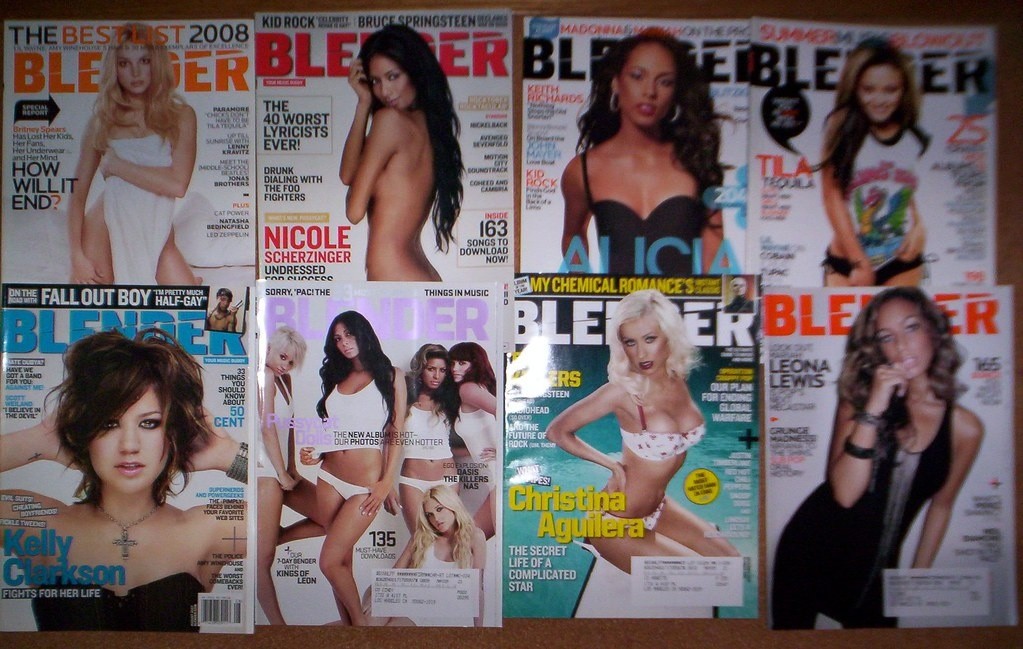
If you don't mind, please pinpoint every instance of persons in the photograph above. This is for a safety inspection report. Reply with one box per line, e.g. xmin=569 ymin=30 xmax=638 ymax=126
xmin=2 ymin=283 xmax=242 ymax=635
xmin=774 ymin=282 xmax=984 ymax=619
xmin=62 ymin=30 xmax=204 ymax=286
xmin=545 ymin=288 xmax=751 ymax=592
xmin=560 ymin=26 xmax=731 ymax=279
xmin=811 ymin=39 xmax=928 ymax=286
xmin=336 ymin=27 xmax=463 ymax=283
xmin=254 ymin=287 xmax=498 ymax=630
